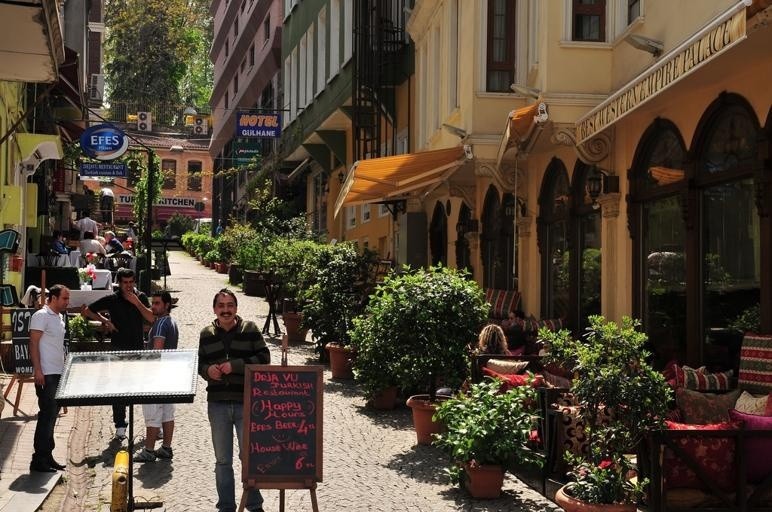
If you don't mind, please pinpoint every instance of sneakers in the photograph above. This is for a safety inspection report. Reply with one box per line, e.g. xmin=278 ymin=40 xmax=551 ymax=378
xmin=115 ymin=428 xmax=126 ymax=438
xmin=154 ymin=446 xmax=172 ymax=458
xmin=133 ymin=449 xmax=156 ymax=462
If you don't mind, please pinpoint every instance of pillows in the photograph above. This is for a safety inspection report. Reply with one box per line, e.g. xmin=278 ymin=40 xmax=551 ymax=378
xmin=660 ymin=421 xmax=745 ymax=485
xmin=485 ymin=359 xmax=530 ymax=374
xmin=734 ymin=390 xmax=772 ymax=416
xmin=675 ymin=364 xmax=735 ymax=394
xmin=549 ymin=401 xmax=614 ymax=465
xmin=727 ymin=406 xmax=772 ymax=481
xmin=738 ymin=331 xmax=772 ymax=395
xmin=484 ymin=287 xmax=523 ymax=318
xmin=676 ymin=386 xmax=741 ymax=425
xmin=521 ymin=317 xmax=565 ymax=336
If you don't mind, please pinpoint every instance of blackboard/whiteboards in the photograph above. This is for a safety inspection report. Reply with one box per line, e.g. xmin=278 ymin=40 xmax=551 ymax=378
xmin=11 ymin=309 xmax=38 ymax=375
xmin=242 ymin=365 xmax=323 ymax=482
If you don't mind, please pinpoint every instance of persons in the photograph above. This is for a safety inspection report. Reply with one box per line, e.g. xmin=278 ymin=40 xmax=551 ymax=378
xmin=132 ymin=289 xmax=179 ymax=463
xmin=198 ymin=288 xmax=270 ymax=512
xmin=29 ymin=284 xmax=70 ymax=472
xmin=505 ymin=310 xmax=524 ymax=324
xmin=84 ymin=266 xmax=164 ymax=439
xmin=47 ymin=185 xmax=136 ymax=265
xmin=476 ymin=324 xmax=512 ymax=356
xmin=500 ymin=321 xmax=526 ymax=355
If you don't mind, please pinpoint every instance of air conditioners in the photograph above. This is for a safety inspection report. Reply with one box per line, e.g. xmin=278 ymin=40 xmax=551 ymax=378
xmin=193 ymin=126 xmax=208 ymax=136
xmin=136 ymin=111 xmax=152 ymax=122
xmin=136 ymin=121 xmax=153 ymax=132
xmin=89 ymin=73 xmax=104 ymax=108
xmin=193 ymin=115 xmax=207 ymax=126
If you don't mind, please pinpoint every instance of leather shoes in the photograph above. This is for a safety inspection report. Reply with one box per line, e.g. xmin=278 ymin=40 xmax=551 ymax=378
xmin=32 ymin=454 xmax=66 ymax=469
xmin=30 ymin=462 xmax=57 ymax=472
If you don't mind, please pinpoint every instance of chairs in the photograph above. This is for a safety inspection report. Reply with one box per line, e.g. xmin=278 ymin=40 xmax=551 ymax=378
xmin=34 ymin=249 xmax=61 ymax=266
xmin=108 ymin=253 xmax=132 ymax=283
xmin=82 ymin=251 xmax=106 ymax=270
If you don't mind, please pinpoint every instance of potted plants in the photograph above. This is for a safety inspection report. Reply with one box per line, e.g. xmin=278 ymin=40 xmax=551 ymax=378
xmin=347 ymin=317 xmax=400 ymax=410
xmin=243 ymin=178 xmax=310 ymax=295
xmin=360 ymin=261 xmax=494 ymax=445
xmin=430 ymin=369 xmax=545 ymax=493
xmin=297 ymin=238 xmax=389 ymax=379
xmin=180 ymin=223 xmax=243 ymax=283
xmin=535 ymin=314 xmax=674 ymax=511
xmin=272 ymin=240 xmax=327 ymax=333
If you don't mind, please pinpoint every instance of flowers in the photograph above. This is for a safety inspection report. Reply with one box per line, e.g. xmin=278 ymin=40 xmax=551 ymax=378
xmin=85 ymin=252 xmax=100 ymax=265
xmin=80 ymin=266 xmax=96 ymax=282
